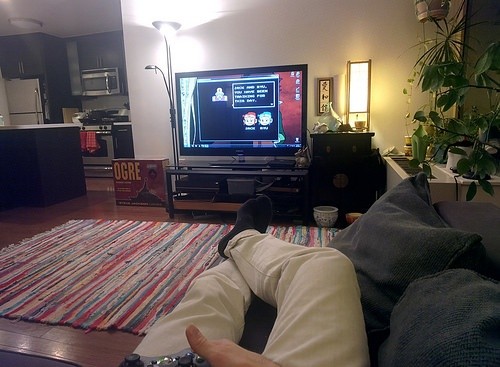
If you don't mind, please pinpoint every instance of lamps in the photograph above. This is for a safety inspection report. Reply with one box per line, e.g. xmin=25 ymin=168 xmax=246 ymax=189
xmin=8 ymin=17 xmax=42 ymax=30
xmin=145 ymin=20 xmax=181 ymax=200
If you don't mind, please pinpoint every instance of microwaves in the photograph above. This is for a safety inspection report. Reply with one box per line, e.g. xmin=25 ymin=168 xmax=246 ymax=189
xmin=81 ymin=68 xmax=120 ymax=96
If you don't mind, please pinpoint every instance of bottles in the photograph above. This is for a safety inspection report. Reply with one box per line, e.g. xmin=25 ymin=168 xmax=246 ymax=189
xmin=0 ymin=114 xmax=5 ymax=125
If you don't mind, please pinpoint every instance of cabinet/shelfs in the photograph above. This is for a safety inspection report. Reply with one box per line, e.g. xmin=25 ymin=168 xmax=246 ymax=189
xmin=309 ymin=131 xmax=375 ymax=212
xmin=166 ymin=160 xmax=311 ymax=225
xmin=0 ymin=32 xmax=128 ymax=124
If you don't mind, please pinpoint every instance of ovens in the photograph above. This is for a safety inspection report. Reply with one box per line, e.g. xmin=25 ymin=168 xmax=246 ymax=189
xmin=80 ymin=132 xmax=115 ymax=166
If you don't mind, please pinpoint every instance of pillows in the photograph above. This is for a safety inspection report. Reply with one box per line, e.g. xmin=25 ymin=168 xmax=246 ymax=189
xmin=324 ymin=171 xmax=483 ymax=344
xmin=373 ymin=268 xmax=500 ymax=367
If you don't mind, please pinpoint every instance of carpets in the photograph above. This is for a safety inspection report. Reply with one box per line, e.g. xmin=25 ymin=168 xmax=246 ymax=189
xmin=0 ymin=219 xmax=343 ymax=355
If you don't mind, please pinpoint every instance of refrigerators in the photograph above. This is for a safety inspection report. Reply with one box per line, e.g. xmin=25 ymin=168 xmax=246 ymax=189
xmin=6 ymin=78 xmax=44 ymax=124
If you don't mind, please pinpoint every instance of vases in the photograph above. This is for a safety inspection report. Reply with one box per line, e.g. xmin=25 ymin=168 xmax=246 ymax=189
xmin=414 ymin=0 xmax=449 ymax=23
xmin=314 ymin=205 xmax=339 ymax=228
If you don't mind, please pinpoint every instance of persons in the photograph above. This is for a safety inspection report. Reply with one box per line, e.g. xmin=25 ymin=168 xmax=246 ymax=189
xmin=121 ymin=195 xmax=370 ymax=367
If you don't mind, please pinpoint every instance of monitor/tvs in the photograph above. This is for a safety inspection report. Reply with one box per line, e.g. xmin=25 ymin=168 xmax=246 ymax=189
xmin=176 ymin=64 xmax=307 ymax=165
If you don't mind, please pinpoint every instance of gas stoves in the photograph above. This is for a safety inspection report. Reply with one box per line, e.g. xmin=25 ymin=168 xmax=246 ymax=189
xmin=78 ymin=115 xmax=129 ymax=124
